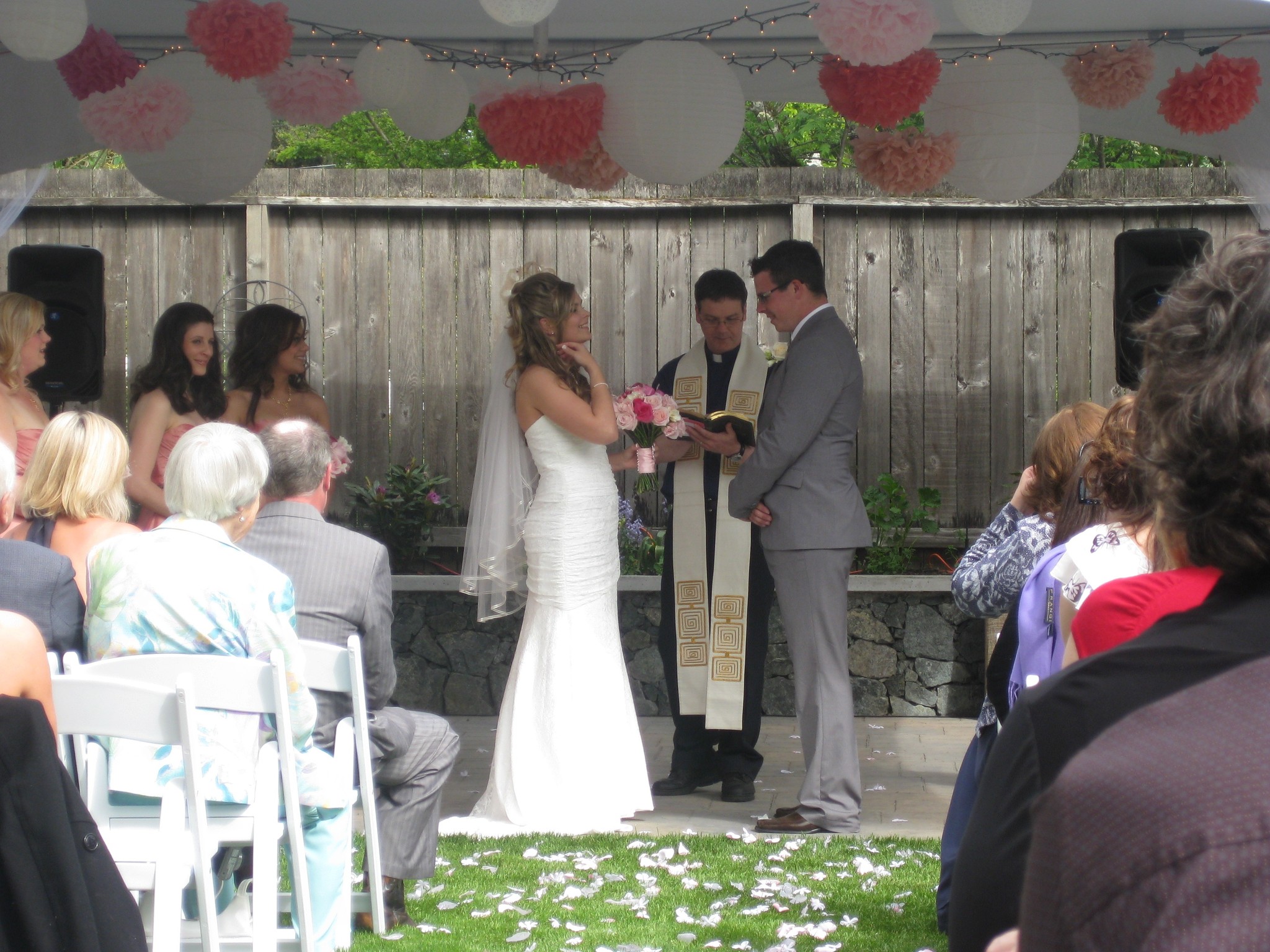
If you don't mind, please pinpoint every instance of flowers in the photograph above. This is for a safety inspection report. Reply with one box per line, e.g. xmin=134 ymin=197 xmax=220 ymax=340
xmin=764 ymin=339 xmax=790 ymax=369
xmin=329 ymin=433 xmax=356 ymax=480
xmin=607 ymin=381 xmax=689 ymax=497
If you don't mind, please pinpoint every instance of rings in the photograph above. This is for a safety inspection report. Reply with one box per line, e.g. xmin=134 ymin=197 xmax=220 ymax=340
xmin=699 ymin=441 xmax=702 ymax=446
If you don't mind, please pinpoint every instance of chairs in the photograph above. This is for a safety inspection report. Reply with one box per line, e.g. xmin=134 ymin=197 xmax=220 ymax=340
xmin=45 ymin=630 xmax=387 ymax=952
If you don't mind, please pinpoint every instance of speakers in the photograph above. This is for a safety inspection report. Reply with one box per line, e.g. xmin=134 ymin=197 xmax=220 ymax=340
xmin=6 ymin=244 xmax=105 ymax=402
xmin=1113 ymin=227 xmax=1213 ymax=391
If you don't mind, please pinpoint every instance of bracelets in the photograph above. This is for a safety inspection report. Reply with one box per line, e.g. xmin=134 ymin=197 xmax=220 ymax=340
xmin=593 ymin=383 xmax=609 ymax=388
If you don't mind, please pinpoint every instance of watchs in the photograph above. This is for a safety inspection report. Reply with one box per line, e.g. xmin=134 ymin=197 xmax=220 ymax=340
xmin=726 ymin=443 xmax=746 ymax=461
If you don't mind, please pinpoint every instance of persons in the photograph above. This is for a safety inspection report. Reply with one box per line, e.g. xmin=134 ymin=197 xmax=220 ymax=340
xmin=84 ymin=422 xmax=352 ymax=951
xmin=121 ymin=303 xmax=230 ymax=531
xmin=0 ymin=410 xmax=140 ymax=752
xmin=437 ymin=270 xmax=657 ymax=841
xmin=218 ymin=303 xmax=330 ymax=438
xmin=726 ymin=239 xmax=875 ymax=834
xmin=1 ymin=290 xmax=52 ymax=482
xmin=235 ymin=418 xmax=460 ymax=935
xmin=936 ymin=233 xmax=1269 ymax=952
xmin=651 ymin=270 xmax=777 ymax=803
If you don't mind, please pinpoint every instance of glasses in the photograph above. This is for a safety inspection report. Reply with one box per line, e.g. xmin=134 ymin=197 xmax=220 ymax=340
xmin=699 ymin=313 xmax=744 ymax=328
xmin=756 ymin=278 xmax=805 ymax=306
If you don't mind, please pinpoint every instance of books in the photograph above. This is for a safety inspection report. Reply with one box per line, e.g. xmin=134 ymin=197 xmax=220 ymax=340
xmin=667 ymin=409 xmax=757 ymax=447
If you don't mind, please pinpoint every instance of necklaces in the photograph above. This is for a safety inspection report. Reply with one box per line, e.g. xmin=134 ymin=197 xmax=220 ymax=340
xmin=185 ymin=390 xmax=191 ymax=402
xmin=570 ymin=371 xmax=579 ymax=386
xmin=269 ymin=384 xmax=291 ymax=416
xmin=26 ymin=389 xmax=39 ymax=411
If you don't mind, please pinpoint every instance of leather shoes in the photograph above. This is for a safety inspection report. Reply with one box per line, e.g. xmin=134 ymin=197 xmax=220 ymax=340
xmin=773 ymin=807 xmax=795 ymax=818
xmin=754 ymin=810 xmax=822 ymax=834
xmin=722 ymin=769 xmax=755 ymax=802
xmin=356 ymin=874 xmax=416 ymax=932
xmin=652 ymin=752 xmax=723 ymax=795
